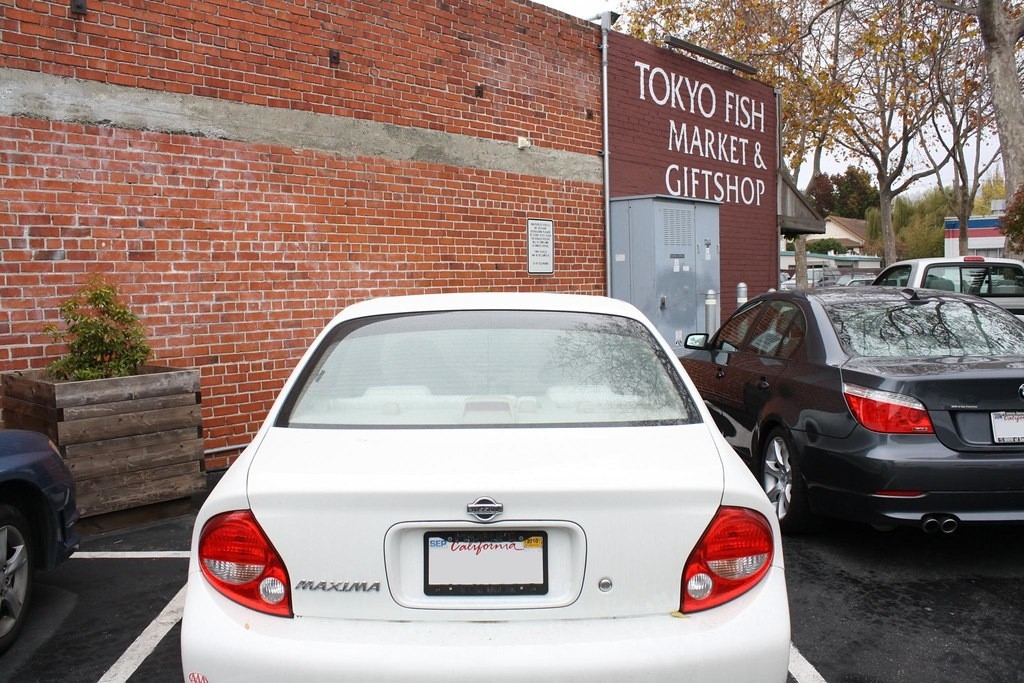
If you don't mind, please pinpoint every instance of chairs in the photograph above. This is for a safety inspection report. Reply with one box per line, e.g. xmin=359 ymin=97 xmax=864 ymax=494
xmin=767 ymin=308 xmax=807 ymax=358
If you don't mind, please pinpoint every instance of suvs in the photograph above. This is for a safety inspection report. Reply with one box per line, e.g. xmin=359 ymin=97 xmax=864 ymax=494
xmin=780 ymin=266 xmax=842 ymax=290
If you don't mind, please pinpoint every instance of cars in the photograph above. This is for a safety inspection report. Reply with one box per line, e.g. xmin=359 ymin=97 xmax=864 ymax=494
xmin=178 ymin=290 xmax=791 ymax=683
xmin=780 ymin=272 xmax=790 ymax=283
xmin=0 ymin=426 xmax=82 ymax=652
xmin=833 ymin=273 xmax=910 ymax=287
xmin=679 ymin=283 xmax=1023 ymax=561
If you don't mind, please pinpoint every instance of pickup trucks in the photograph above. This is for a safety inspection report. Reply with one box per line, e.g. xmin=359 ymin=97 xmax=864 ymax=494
xmin=868 ymin=257 xmax=1024 ymax=324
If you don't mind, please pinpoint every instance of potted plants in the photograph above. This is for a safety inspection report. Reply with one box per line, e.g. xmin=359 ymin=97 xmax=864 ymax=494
xmin=0 ymin=271 xmax=210 ymax=521
